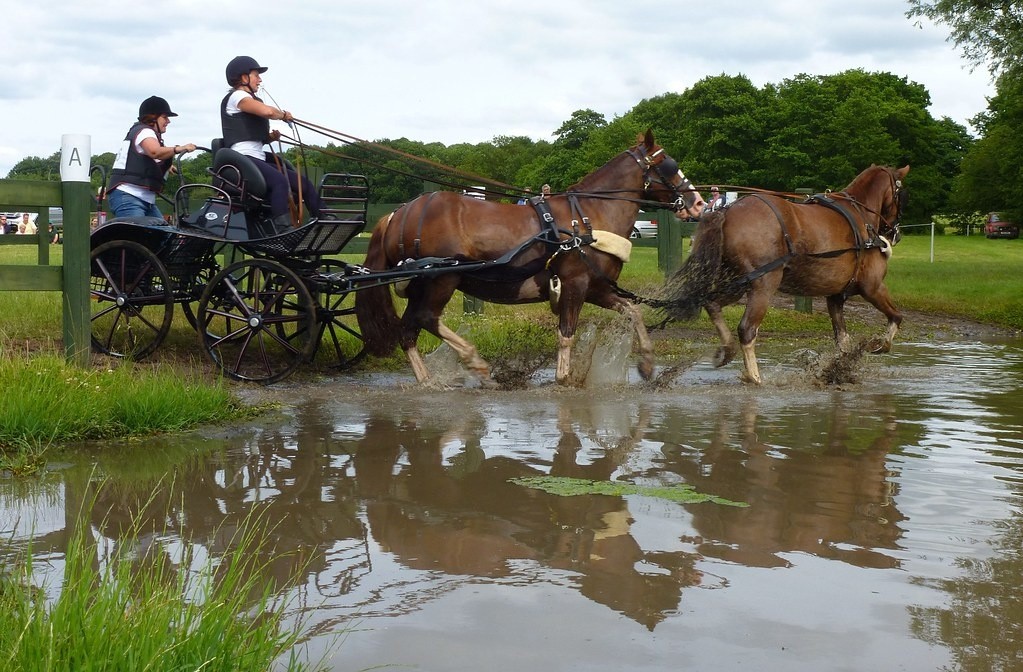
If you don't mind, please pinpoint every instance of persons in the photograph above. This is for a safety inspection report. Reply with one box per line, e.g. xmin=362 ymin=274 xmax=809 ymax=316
xmin=0 ymin=211 xmax=100 ymax=244
xmin=705 ymin=186 xmax=726 ymax=213
xmin=516 ymin=183 xmax=551 ymax=206
xmin=220 ymin=54 xmax=366 ymax=249
xmin=105 ymin=96 xmax=198 ymax=297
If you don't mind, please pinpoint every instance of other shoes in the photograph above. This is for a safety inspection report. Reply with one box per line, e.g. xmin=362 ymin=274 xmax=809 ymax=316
xmin=134 ymin=282 xmax=165 ymax=296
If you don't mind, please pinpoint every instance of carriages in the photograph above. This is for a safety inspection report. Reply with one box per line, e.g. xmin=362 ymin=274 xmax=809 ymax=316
xmin=89 ymin=126 xmax=911 ymax=391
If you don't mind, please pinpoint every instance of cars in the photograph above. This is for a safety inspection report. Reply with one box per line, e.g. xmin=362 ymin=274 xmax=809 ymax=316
xmin=983 ymin=212 xmax=1020 ymax=239
xmin=0 ymin=207 xmax=64 ymax=244
xmin=628 ymin=209 xmax=658 ymax=239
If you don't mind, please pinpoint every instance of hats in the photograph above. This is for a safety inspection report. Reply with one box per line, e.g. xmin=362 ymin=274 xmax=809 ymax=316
xmin=49 ymin=219 xmax=53 ymax=224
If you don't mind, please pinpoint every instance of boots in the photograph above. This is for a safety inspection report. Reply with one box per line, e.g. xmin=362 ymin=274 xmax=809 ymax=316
xmin=320 ymin=214 xmax=363 ymax=221
xmin=274 ymin=214 xmax=298 ymax=233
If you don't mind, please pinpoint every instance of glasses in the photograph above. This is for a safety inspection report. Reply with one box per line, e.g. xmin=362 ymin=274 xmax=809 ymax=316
xmin=1 ymin=219 xmax=6 ymax=220
xmin=49 ymin=223 xmax=52 ymax=224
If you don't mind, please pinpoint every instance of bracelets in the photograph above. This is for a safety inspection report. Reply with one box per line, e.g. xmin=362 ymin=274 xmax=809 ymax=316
xmin=282 ymin=109 xmax=288 ymax=120
xmin=173 ymin=144 xmax=180 ymax=155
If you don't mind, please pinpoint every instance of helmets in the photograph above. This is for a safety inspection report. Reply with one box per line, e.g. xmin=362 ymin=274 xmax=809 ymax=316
xmin=139 ymin=95 xmax=178 ymax=117
xmin=226 ymin=56 xmax=268 ymax=85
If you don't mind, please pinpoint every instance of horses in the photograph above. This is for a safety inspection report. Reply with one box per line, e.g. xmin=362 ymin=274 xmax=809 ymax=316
xmin=356 ymin=128 xmax=707 ymax=388
xmin=662 ymin=163 xmax=910 ymax=387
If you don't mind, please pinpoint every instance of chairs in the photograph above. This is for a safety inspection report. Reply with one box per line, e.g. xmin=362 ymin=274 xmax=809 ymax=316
xmin=205 ymin=138 xmax=298 ymax=208
